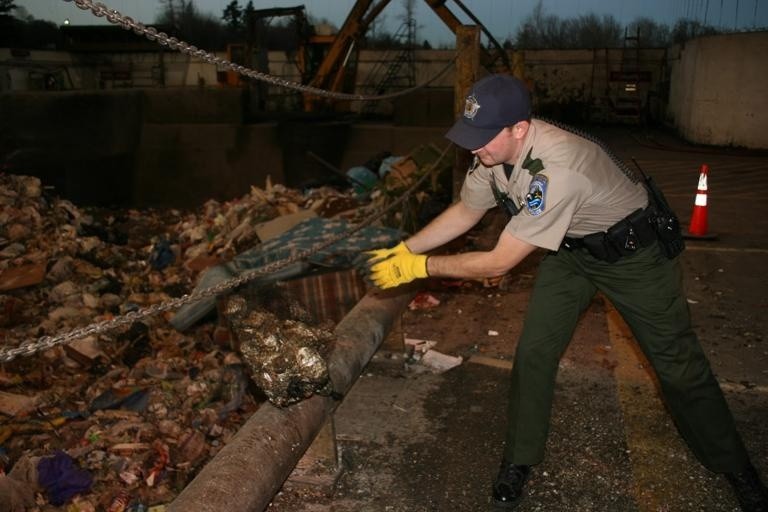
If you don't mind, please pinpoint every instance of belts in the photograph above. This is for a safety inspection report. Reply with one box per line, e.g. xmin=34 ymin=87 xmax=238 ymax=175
xmin=559 ymin=236 xmax=585 ymax=252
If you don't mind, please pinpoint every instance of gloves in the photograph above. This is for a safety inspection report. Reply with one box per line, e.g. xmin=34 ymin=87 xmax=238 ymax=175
xmin=352 ymin=239 xmax=430 ymax=298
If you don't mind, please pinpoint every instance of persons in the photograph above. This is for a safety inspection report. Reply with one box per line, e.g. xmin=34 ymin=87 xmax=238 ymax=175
xmin=351 ymin=71 xmax=768 ymax=511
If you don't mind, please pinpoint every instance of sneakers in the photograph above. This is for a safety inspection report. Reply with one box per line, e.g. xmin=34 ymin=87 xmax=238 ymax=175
xmin=724 ymin=459 xmax=767 ymax=512
xmin=492 ymin=456 xmax=532 ymax=507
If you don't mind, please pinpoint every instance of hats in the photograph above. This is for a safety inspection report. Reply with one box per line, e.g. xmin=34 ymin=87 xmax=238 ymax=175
xmin=443 ymin=72 xmax=533 ymax=151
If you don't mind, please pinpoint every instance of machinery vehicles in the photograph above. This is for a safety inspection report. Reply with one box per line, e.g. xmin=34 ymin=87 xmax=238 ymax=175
xmin=214 ymin=1 xmax=538 ymax=135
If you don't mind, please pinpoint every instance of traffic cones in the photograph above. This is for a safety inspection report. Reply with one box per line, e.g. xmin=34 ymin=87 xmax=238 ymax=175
xmin=680 ymin=163 xmax=719 ymax=242
